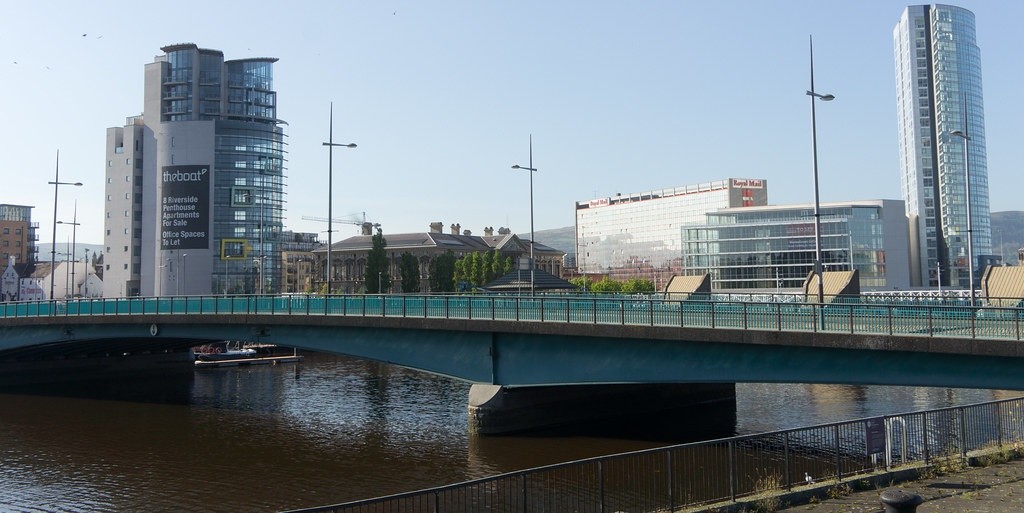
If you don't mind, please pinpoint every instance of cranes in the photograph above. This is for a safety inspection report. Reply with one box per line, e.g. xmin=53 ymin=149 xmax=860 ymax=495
xmin=302 ymin=211 xmax=381 ymax=235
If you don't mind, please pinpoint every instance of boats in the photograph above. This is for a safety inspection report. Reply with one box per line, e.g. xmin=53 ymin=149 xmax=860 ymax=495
xmin=193 ymin=341 xmax=257 ymax=360
xmin=243 ymin=341 xmax=277 ymax=354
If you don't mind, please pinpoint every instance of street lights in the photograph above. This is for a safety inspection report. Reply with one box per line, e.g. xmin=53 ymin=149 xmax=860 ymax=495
xmin=50 ymin=182 xmax=83 ymax=301
xmin=226 ymin=255 xmax=230 ymax=296
xmin=951 ymin=129 xmax=977 ymax=314
xmin=997 ymin=230 xmax=1004 ymax=265
xmin=810 ymin=92 xmax=835 ymax=329
xmin=184 ymin=253 xmax=187 ymax=296
xmin=243 ymin=193 xmax=264 ymax=297
xmin=166 ymin=258 xmax=179 ymax=297
xmin=297 ymin=259 xmax=304 ymax=294
xmin=511 ymin=164 xmax=535 ymax=306
xmin=56 ymin=220 xmax=74 ymax=301
xmin=329 ymin=143 xmax=357 ymax=315
xmin=159 ymin=265 xmax=167 ymax=298
xmin=84 ymin=249 xmax=90 ymax=301
xmin=378 ymin=272 xmax=382 ymax=294
